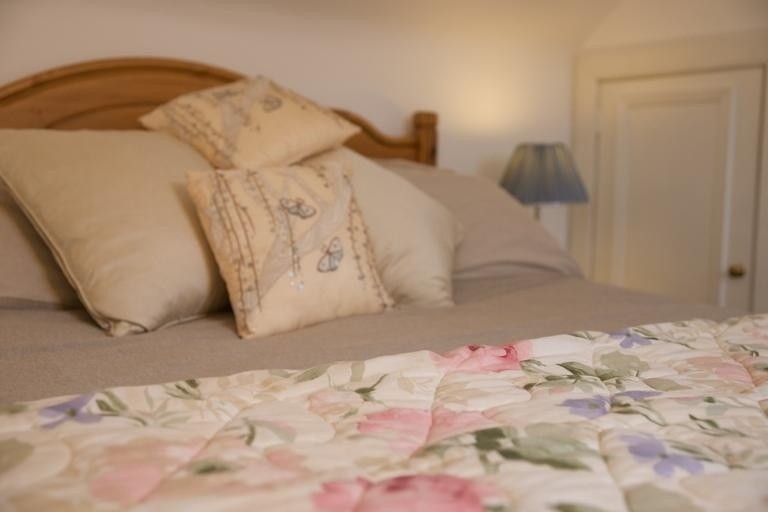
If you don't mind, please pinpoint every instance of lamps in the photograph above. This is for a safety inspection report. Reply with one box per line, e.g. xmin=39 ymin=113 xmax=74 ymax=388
xmin=501 ymin=140 xmax=592 ymax=223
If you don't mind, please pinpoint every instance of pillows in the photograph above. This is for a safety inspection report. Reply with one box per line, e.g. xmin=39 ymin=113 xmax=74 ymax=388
xmin=299 ymin=146 xmax=469 ymax=308
xmin=0 ymin=129 xmax=228 ymax=339
xmin=0 ymin=188 xmax=79 ymax=306
xmin=184 ymin=167 xmax=390 ymax=338
xmin=367 ymin=157 xmax=575 ymax=280
xmin=136 ymin=78 xmax=362 ymax=169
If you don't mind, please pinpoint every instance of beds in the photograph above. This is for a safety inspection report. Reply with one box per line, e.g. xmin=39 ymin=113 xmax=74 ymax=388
xmin=0 ymin=56 xmax=768 ymax=512
